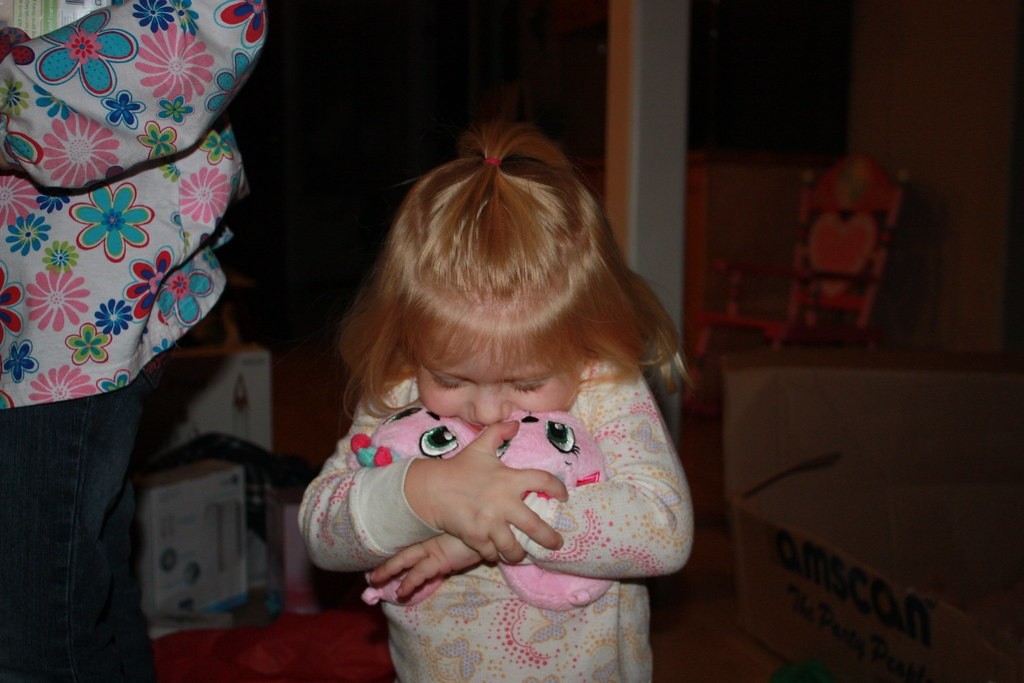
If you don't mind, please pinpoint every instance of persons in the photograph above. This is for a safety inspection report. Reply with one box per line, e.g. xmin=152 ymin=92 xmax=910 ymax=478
xmin=299 ymin=120 xmax=694 ymax=683
xmin=0 ymin=0 xmax=267 ymax=683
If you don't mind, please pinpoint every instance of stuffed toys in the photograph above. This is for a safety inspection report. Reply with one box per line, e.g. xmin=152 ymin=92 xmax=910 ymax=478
xmin=351 ymin=408 xmax=615 ymax=610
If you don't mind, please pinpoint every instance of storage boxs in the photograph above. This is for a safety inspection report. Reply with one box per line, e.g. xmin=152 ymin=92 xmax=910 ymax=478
xmin=720 ymin=368 xmax=1024 ymax=682
xmin=135 ymin=344 xmax=274 ymax=458
xmin=133 ymin=459 xmax=247 ymax=614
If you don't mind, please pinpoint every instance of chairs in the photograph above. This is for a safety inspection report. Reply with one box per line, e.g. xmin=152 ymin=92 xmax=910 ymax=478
xmin=682 ymin=179 xmax=907 ymax=416
